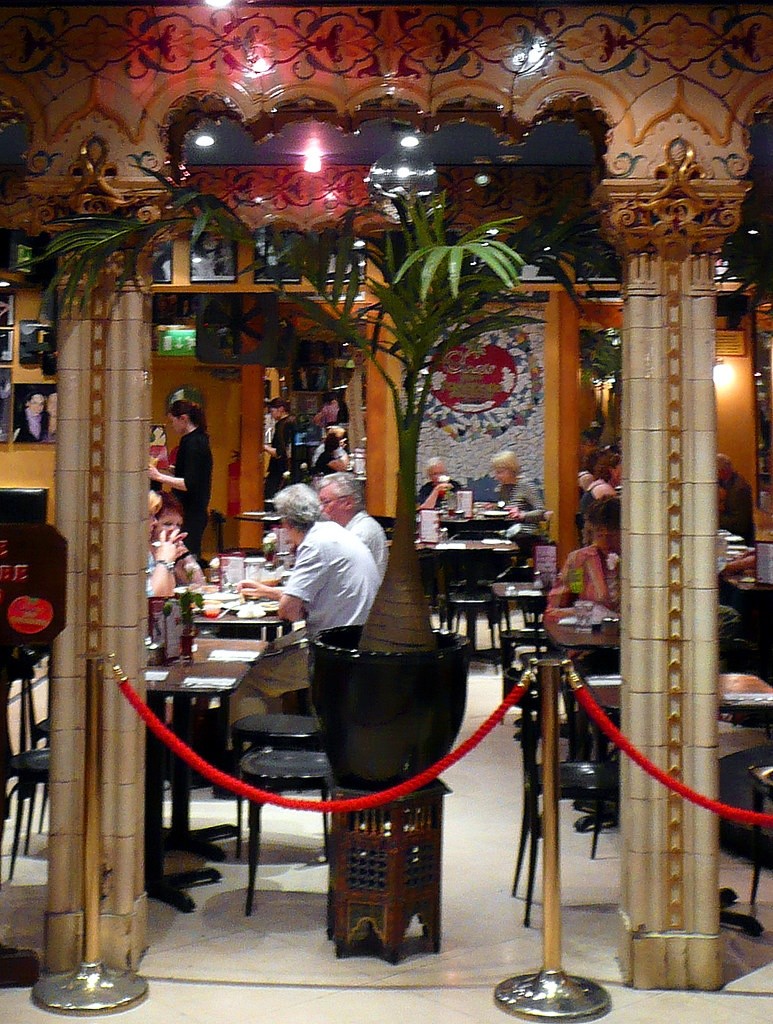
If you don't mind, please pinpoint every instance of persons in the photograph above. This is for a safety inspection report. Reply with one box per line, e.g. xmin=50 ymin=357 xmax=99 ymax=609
xmin=147 ymin=399 xmax=213 ymax=563
xmin=213 ymin=482 xmax=383 ymax=797
xmin=716 ymin=454 xmax=760 ymax=652
xmin=263 ymin=388 xmax=351 ymax=534
xmin=146 ymin=489 xmax=212 ymax=730
xmin=545 ymin=443 xmax=621 ymax=798
xmin=14 ymin=394 xmax=50 ymax=444
xmin=417 ymin=456 xmax=463 ymax=609
xmin=482 ymin=451 xmax=547 ymax=582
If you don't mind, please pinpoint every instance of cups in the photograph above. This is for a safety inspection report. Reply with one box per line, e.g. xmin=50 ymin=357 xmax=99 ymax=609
xmin=574 ymin=600 xmax=594 ymax=633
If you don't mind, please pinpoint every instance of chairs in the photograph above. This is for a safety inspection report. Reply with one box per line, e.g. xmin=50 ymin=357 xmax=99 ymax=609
xmin=748 ymin=758 xmax=773 ymax=910
xmin=511 ymin=711 xmax=615 ymax=932
xmin=433 ymin=547 xmax=505 ymax=675
xmin=0 ymin=652 xmax=49 ymax=879
xmin=24 ymin=636 xmax=50 ymax=836
xmin=213 ymin=509 xmax=262 ymax=556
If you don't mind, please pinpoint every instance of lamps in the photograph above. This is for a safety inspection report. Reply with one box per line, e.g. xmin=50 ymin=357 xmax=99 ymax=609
xmin=190 ymin=126 xmax=220 ymax=149
xmin=266 ymin=123 xmax=355 ymax=174
xmin=474 ymin=169 xmax=493 ymax=187
xmin=396 ymin=125 xmax=427 ymax=151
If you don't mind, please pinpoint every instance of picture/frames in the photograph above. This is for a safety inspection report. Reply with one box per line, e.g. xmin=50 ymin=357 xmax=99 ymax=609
xmin=0 ymin=293 xmax=17 ymax=443
xmin=253 ymin=229 xmax=304 ymax=284
xmin=186 ymin=232 xmax=239 ymax=284
xmin=514 ymin=249 xmax=561 ymax=283
xmin=12 ymin=383 xmax=56 ymax=444
xmin=153 ymin=238 xmax=173 ymax=283
xmin=572 ymin=226 xmax=619 ymax=284
xmin=325 ymin=240 xmax=370 ymax=284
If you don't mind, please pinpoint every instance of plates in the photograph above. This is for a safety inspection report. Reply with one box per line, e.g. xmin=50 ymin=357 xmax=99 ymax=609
xmin=483 ymin=510 xmax=509 ymax=516
xmin=172 ymin=586 xmax=280 ymax=613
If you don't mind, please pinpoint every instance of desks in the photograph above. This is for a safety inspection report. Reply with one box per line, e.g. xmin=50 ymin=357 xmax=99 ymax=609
xmin=190 ymin=582 xmax=293 ymax=639
xmin=584 ymin=667 xmax=773 ymax=715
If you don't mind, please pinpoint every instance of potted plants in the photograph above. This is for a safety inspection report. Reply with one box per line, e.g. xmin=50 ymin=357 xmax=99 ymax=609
xmin=6 ymin=157 xmax=594 ymax=794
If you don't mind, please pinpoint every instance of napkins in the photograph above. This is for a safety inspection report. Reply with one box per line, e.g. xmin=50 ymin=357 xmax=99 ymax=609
xmin=482 ymin=537 xmax=512 ymax=546
xmin=435 ymin=540 xmax=466 ymax=551
xmin=520 ymin=588 xmax=543 ymax=598
xmin=147 ymin=670 xmax=168 ymax=681
xmin=262 ymin=516 xmax=282 ymax=521
xmin=556 ymin=615 xmax=578 ymax=626
xmin=727 ymin=535 xmax=742 ymax=541
xmin=183 ymin=673 xmax=236 ymax=690
xmin=242 ymin=510 xmax=266 ymax=516
xmin=719 ymin=530 xmax=731 ymax=536
xmin=207 ymin=648 xmax=262 ymax=663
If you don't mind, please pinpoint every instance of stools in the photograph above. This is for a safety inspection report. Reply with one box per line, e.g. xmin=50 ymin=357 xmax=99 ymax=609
xmin=228 ymin=709 xmax=344 ymax=862
xmin=230 ymin=735 xmax=335 ymax=915
xmin=323 ymin=777 xmax=455 ymax=969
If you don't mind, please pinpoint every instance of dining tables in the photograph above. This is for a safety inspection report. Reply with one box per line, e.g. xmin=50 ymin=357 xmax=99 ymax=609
xmin=540 ymin=601 xmax=620 ymax=652
xmin=718 ymin=528 xmax=745 ymax=542
xmin=489 ymin=573 xmax=550 ymax=606
xmin=147 ymin=629 xmax=268 ymax=916
xmin=439 ymin=501 xmax=510 ymax=526
xmin=724 ymin=551 xmax=773 ymax=593
xmin=417 ymin=533 xmax=524 ymax=661
xmin=231 ymin=506 xmax=285 ymax=524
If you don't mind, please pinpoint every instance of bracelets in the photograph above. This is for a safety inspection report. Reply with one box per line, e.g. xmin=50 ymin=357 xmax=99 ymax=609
xmin=176 ymin=550 xmax=191 ymax=561
xmin=156 ymin=559 xmax=174 ymax=569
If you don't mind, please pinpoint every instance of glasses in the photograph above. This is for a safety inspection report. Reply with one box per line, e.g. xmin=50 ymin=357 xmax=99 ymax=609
xmin=323 ymin=494 xmax=352 ymax=505
